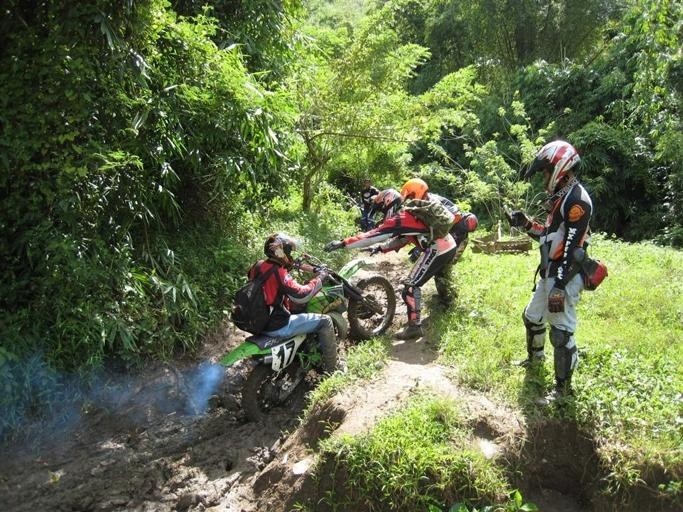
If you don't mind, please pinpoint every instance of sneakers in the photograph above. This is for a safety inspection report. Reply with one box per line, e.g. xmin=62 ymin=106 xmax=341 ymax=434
xmin=518 ymin=357 xmax=544 ymax=367
xmin=535 ymin=387 xmax=573 ymax=406
xmin=432 ymin=294 xmax=449 ymax=308
xmin=393 ymin=326 xmax=422 ymax=340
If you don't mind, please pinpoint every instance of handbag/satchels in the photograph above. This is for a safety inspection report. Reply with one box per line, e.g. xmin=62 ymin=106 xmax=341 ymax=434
xmin=461 ymin=212 xmax=477 ymax=232
xmin=579 ymin=257 xmax=608 ymax=291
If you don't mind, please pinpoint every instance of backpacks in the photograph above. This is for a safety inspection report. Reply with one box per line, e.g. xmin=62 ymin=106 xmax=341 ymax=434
xmin=230 ymin=260 xmax=281 ymax=335
xmin=396 ymin=198 xmax=455 ymax=246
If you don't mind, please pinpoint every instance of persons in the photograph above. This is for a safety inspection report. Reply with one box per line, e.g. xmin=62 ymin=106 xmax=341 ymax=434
xmin=502 ymin=140 xmax=608 ymax=410
xmin=400 ymin=178 xmax=478 ymax=309
xmin=323 ymin=189 xmax=457 ymax=341
xmin=360 ymin=179 xmax=382 ymax=231
xmin=247 ymin=233 xmax=348 ymax=378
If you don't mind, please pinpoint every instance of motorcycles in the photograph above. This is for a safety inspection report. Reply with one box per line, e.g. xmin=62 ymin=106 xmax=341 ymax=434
xmin=218 ymin=252 xmax=396 ymax=424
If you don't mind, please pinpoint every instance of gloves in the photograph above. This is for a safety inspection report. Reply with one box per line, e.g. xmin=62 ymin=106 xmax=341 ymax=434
xmin=317 ymin=268 xmax=334 ymax=285
xmin=360 ymin=245 xmax=382 ymax=256
xmin=548 ymin=288 xmax=565 ymax=313
xmin=295 ymin=253 xmax=311 ymax=265
xmin=408 ymin=246 xmax=422 ymax=263
xmin=503 ymin=205 xmax=532 ymax=233
xmin=323 ymin=238 xmax=345 ymax=253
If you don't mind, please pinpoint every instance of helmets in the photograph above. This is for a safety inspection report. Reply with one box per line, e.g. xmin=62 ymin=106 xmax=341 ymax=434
xmin=518 ymin=140 xmax=581 ymax=195
xmin=264 ymin=233 xmax=296 ymax=266
xmin=369 ymin=178 xmax=429 ymax=219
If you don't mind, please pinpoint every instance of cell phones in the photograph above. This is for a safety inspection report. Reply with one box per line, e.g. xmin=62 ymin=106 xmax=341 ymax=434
xmin=503 ymin=204 xmax=513 ymax=220
